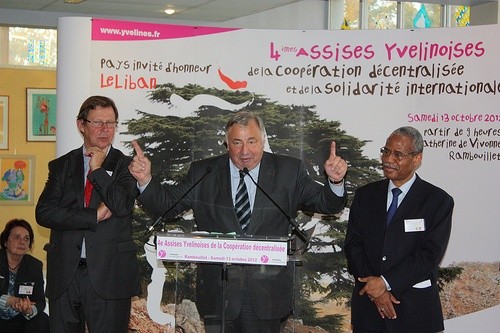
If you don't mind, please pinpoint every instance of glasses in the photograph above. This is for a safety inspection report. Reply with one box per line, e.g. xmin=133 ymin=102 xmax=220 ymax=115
xmin=83 ymin=118 xmax=118 ymax=128
xmin=380 ymin=146 xmax=420 ymax=160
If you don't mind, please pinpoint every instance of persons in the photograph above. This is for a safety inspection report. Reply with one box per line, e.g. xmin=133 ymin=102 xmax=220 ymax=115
xmin=35 ymin=96 xmax=138 ymax=333
xmin=128 ymin=112 xmax=348 ymax=333
xmin=344 ymin=124 xmax=455 ymax=333
xmin=0 ymin=219 xmax=50 ymax=333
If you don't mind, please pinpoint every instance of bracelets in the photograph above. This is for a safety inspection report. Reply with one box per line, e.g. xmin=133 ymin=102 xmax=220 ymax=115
xmin=328 ymin=178 xmax=343 ymax=184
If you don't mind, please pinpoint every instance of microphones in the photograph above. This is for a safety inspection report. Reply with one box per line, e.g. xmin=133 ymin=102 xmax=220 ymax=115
xmin=243 ymin=166 xmax=307 ymax=236
xmin=143 ymin=167 xmax=212 ymax=236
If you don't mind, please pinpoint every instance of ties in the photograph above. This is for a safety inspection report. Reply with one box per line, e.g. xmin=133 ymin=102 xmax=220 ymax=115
xmin=83 ymin=169 xmax=94 ymax=209
xmin=386 ymin=187 xmax=402 ymax=228
xmin=235 ymin=170 xmax=252 ymax=233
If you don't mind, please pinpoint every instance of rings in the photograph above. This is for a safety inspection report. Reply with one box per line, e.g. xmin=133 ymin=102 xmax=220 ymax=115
xmin=380 ymin=308 xmax=384 ymax=312
xmin=369 ymin=296 xmax=371 ymax=298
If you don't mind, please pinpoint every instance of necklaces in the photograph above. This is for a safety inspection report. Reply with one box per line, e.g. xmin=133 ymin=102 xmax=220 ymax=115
xmin=9 ymin=264 xmax=19 ymax=270
